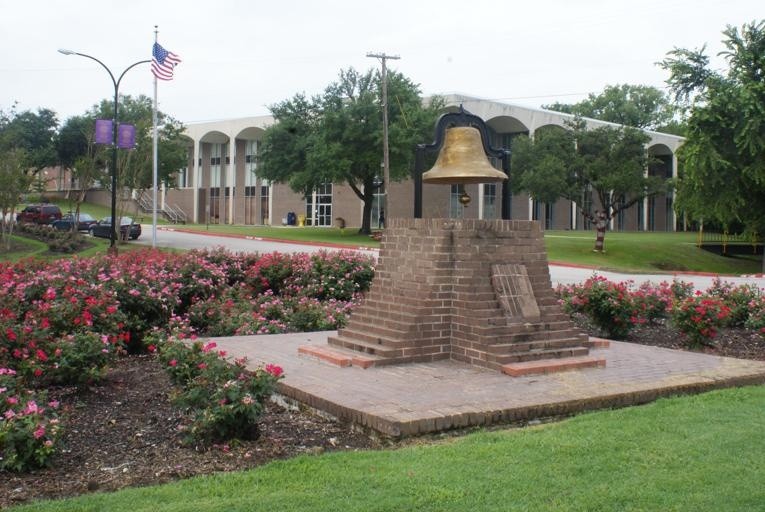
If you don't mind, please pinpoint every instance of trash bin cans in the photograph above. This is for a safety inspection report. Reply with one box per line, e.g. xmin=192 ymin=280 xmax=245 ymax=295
xmin=288 ymin=212 xmax=295 ymax=225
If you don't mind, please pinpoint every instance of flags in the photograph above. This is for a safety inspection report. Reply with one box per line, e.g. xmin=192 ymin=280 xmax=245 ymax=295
xmin=150 ymin=43 xmax=182 ymax=82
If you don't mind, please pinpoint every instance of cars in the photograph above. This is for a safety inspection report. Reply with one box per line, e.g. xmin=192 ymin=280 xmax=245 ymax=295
xmin=53 ymin=212 xmax=97 ymax=231
xmin=16 ymin=205 xmax=62 ymax=226
xmin=88 ymin=216 xmax=140 ymax=239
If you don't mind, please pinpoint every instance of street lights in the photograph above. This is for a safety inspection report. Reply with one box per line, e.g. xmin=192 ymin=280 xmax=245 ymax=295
xmin=58 ymin=48 xmax=152 ymax=250
